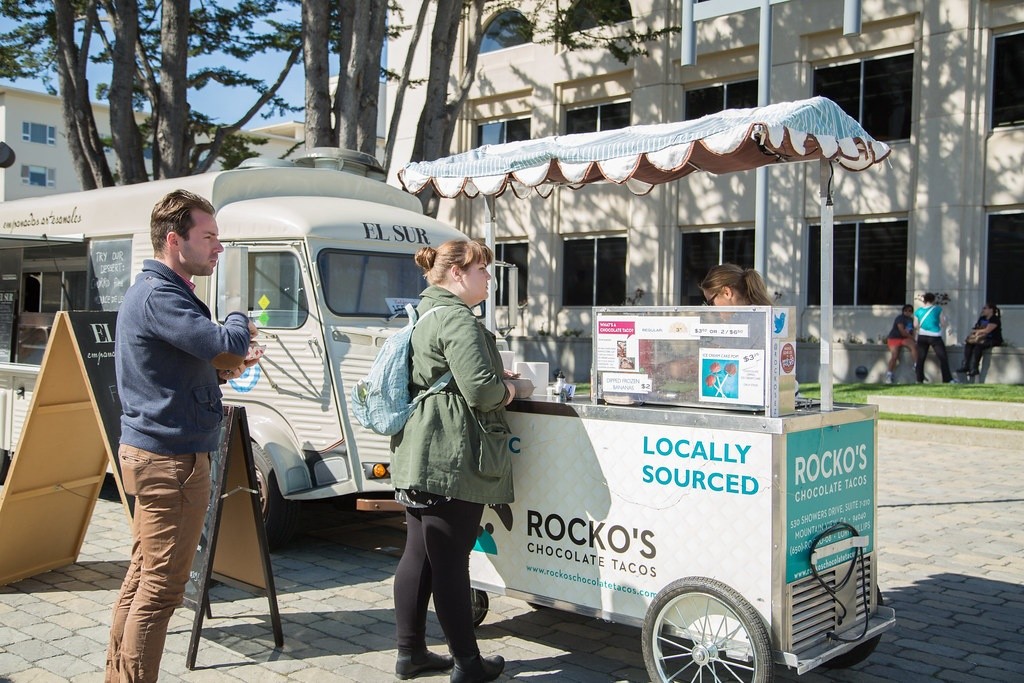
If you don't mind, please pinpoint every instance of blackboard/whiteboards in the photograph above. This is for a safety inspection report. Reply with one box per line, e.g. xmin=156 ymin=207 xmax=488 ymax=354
xmin=1 ymin=310 xmax=220 ymax=593
xmin=86 ymin=238 xmax=132 ymax=312
xmin=181 ymin=404 xmax=273 ymax=612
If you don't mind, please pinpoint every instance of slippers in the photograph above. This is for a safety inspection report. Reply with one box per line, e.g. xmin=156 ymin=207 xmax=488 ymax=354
xmin=450 ymin=654 xmax=505 ymax=683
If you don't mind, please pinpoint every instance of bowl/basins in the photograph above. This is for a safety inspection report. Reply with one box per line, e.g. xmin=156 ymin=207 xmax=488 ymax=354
xmin=505 ymin=377 xmax=536 ymax=399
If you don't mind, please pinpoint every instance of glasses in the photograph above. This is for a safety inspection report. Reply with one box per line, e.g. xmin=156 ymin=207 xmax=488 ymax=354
xmin=703 ymin=293 xmax=719 ymax=307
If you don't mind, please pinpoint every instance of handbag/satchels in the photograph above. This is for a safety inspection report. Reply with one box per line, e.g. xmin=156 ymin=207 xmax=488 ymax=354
xmin=967 ymin=326 xmax=985 ymax=344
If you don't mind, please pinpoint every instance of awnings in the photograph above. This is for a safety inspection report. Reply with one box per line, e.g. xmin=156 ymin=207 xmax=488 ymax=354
xmin=397 ymin=96 xmax=890 ymax=197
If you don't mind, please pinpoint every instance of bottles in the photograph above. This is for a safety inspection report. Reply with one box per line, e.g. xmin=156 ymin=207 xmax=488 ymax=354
xmin=556 ymin=371 xmax=566 ymax=403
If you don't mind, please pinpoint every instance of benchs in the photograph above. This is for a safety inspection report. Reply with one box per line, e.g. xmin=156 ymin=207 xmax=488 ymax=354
xmin=795 ymin=344 xmax=1024 ymax=384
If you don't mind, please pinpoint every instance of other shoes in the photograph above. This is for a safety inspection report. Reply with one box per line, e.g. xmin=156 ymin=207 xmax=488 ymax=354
xmin=969 ymin=368 xmax=979 ymax=376
xmin=949 ymin=378 xmax=957 ymax=384
xmin=957 ymin=366 xmax=968 ymax=372
xmin=886 ymin=375 xmax=892 ymax=384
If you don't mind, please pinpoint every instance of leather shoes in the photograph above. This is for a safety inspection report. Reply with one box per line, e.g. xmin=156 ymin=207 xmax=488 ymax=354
xmin=395 ymin=648 xmax=456 ymax=680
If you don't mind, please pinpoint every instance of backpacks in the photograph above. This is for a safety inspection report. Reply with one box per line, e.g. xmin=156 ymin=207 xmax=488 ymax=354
xmin=351 ymin=303 xmax=455 ymax=436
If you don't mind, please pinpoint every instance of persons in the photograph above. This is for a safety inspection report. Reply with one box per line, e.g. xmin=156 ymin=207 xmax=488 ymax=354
xmin=103 ymin=188 xmax=258 ymax=683
xmin=391 ymin=240 xmax=521 ymax=683
xmin=884 ymin=304 xmax=933 ymax=384
xmin=956 ymin=302 xmax=1002 ymax=377
xmin=656 ymin=263 xmax=800 ymax=402
xmin=914 ymin=292 xmax=959 ymax=385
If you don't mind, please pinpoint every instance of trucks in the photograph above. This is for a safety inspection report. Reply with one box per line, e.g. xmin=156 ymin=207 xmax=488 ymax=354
xmin=0 ymin=147 xmax=492 ymax=551
